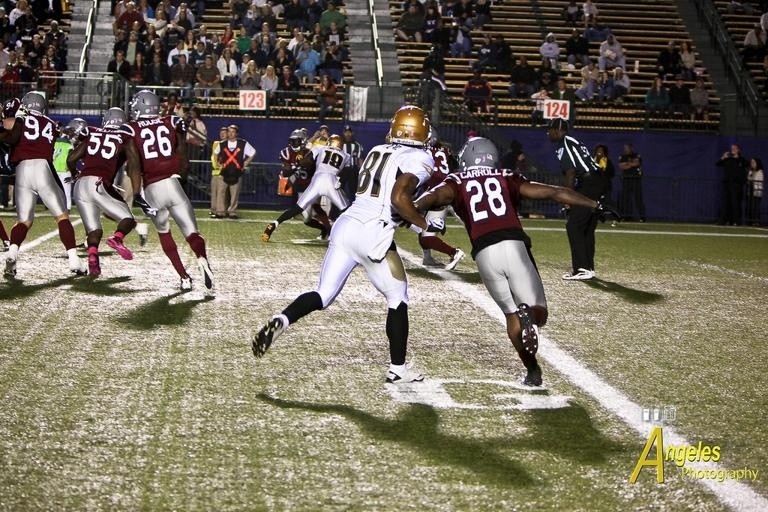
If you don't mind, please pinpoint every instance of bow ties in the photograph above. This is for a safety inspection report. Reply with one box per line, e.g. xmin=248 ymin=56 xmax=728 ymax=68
xmin=516 ymin=302 xmax=538 ymax=356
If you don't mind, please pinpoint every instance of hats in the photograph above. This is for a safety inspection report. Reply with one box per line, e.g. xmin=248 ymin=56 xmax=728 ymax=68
xmin=543 ymin=118 xmax=569 ymax=133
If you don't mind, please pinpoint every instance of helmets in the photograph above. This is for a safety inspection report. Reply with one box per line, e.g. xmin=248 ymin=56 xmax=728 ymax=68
xmin=0 ymin=91 xmax=160 ymax=139
xmin=456 ymin=136 xmax=499 ymax=173
xmin=388 ymin=105 xmax=432 ymax=147
xmin=289 ymin=125 xmax=352 ymax=150
xmin=428 ymin=123 xmax=439 ymax=148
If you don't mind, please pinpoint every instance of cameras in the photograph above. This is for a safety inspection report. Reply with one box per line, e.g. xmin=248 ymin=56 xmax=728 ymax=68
xmin=727 ymin=153 xmax=735 ymax=157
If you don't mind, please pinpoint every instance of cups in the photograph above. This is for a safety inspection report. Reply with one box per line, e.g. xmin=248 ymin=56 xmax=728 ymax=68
xmin=634 ymin=61 xmax=639 ymax=73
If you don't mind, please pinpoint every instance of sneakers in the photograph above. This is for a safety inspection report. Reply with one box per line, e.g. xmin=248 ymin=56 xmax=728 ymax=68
xmin=67 ymin=255 xmax=88 ymax=277
xmin=209 ymin=214 xmax=225 ymax=218
xmin=423 ymin=256 xmax=444 ymax=266
xmin=561 ymin=267 xmax=593 ymax=280
xmin=228 ymin=214 xmax=237 ymax=220
xmin=261 ymin=223 xmax=276 ymax=242
xmin=316 ymin=225 xmax=331 ymax=242
xmin=197 ymin=256 xmax=214 ymax=289
xmin=524 ymin=369 xmax=543 ymax=386
xmin=138 ymin=223 xmax=149 ymax=246
xmin=2 ymin=240 xmax=11 ymax=250
xmin=385 ymin=368 xmax=425 ymax=383
xmin=180 ymin=274 xmax=193 ymax=291
xmin=251 ymin=314 xmax=287 ymax=360
xmin=445 ymin=247 xmax=466 ymax=271
xmin=106 ymin=234 xmax=133 ymax=261
xmin=88 ymin=252 xmax=101 ymax=277
xmin=3 ymin=254 xmax=17 ymax=281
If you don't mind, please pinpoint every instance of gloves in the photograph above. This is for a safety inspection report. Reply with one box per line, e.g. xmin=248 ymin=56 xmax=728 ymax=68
xmin=424 ymin=217 xmax=446 ymax=236
xmin=590 ymin=199 xmax=622 ymax=224
xmin=391 ymin=201 xmax=424 ymax=229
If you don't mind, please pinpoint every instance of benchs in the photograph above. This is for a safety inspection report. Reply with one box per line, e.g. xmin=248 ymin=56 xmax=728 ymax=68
xmin=0 ymin=0 xmax=766 ymax=122
